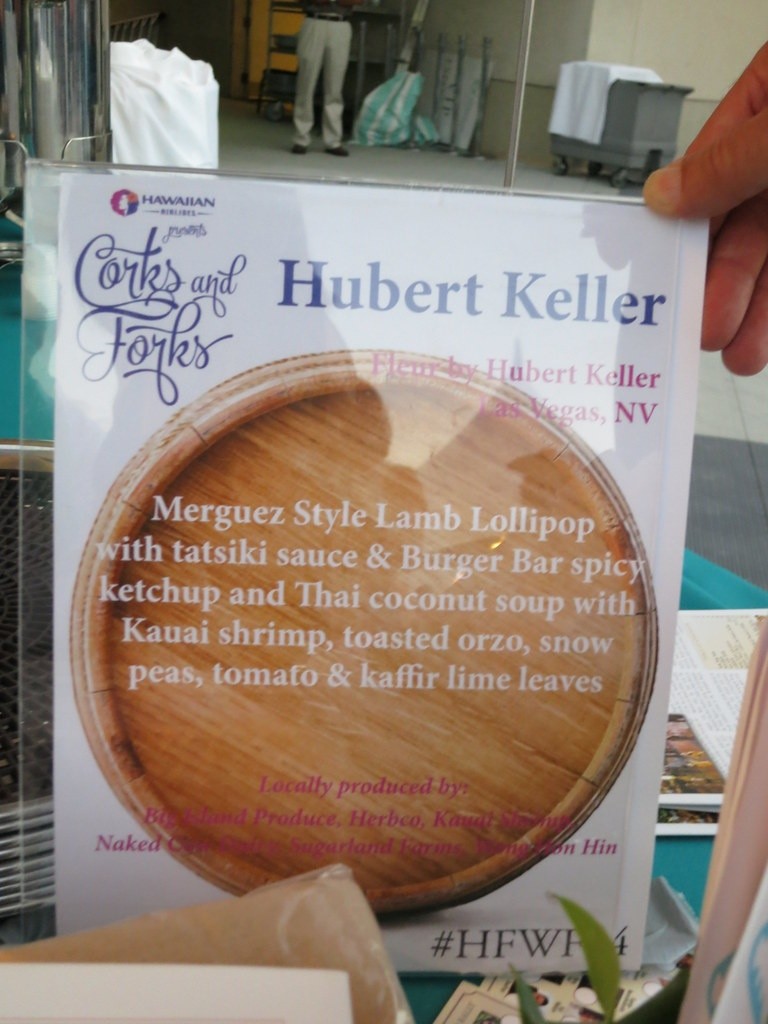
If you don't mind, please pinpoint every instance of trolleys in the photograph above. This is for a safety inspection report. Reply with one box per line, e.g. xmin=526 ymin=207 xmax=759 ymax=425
xmin=255 ymin=0 xmax=401 ymax=141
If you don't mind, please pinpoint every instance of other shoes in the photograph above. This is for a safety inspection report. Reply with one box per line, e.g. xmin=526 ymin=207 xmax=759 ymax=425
xmin=293 ymin=144 xmax=305 ymax=154
xmin=326 ymin=147 xmax=348 ymax=157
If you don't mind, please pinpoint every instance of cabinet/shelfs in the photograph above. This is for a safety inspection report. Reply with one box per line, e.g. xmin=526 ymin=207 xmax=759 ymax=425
xmin=255 ymin=0 xmax=401 ymax=138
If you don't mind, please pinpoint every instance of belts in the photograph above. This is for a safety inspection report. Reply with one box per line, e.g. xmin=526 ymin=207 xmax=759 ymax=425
xmin=306 ymin=14 xmax=347 ymax=21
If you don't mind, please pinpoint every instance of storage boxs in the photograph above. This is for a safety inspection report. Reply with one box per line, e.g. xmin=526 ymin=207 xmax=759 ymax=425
xmin=1 ymin=866 xmax=422 ymax=1023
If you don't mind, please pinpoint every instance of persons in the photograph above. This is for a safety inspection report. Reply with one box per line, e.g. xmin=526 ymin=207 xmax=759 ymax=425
xmin=644 ymin=44 xmax=768 ymax=376
xmin=291 ymin=1 xmax=353 ymax=156
